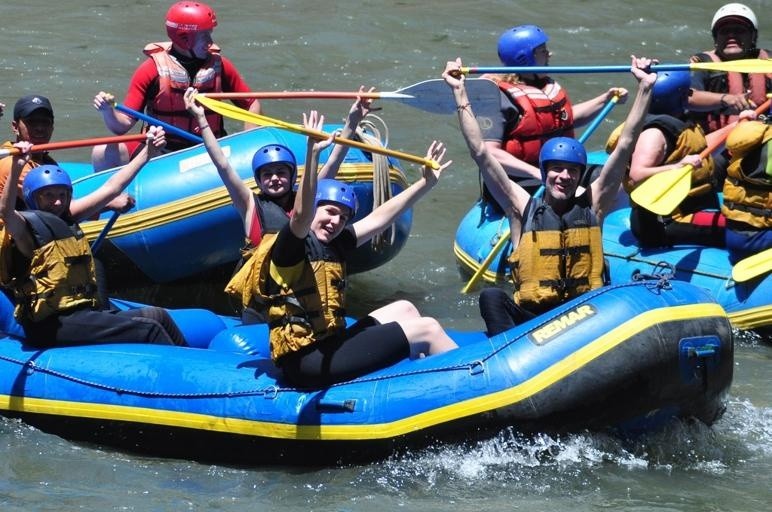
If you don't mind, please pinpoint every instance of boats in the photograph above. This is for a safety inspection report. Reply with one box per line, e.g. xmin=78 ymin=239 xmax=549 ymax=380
xmin=0 ymin=282 xmax=731 ymax=471
xmin=453 ymin=150 xmax=772 ymax=345
xmin=1 ymin=121 xmax=419 ymax=299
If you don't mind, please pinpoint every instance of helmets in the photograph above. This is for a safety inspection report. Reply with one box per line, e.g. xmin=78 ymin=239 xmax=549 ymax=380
xmin=497 ymin=26 xmax=549 ymax=73
xmin=252 ymin=144 xmax=296 ymax=194
xmin=539 ymin=136 xmax=587 ymax=185
xmin=650 ymin=70 xmax=691 ymax=116
xmin=22 ymin=164 xmax=73 ymax=219
xmin=710 ymin=2 xmax=759 ymax=47
xmin=314 ymin=178 xmax=360 ymax=221
xmin=165 ymin=1 xmax=218 ymax=51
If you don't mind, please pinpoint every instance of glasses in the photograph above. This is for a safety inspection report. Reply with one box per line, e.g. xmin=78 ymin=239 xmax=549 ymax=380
xmin=26 ymin=117 xmax=52 ymax=127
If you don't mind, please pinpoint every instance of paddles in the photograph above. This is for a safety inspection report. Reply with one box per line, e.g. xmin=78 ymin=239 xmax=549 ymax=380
xmin=193 ymin=92 xmax=442 ymax=174
xmin=631 ymin=94 xmax=771 ymax=217
xmin=460 ymin=57 xmax=772 ymax=74
xmin=459 ymin=96 xmax=621 ymax=293
xmin=206 ymin=89 xmax=415 ymax=100
xmin=732 ymin=245 xmax=772 ymax=284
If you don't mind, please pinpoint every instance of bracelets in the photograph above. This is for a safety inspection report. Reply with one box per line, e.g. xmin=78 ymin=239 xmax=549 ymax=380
xmin=457 ymin=103 xmax=471 ymax=110
xmin=200 ymin=123 xmax=209 ymax=131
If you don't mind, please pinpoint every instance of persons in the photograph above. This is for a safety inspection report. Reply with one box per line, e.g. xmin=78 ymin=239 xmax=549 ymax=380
xmin=222 ymin=111 xmax=460 ymax=389
xmin=183 ymin=86 xmax=382 ymax=326
xmin=626 ymin=0 xmax=771 ymax=262
xmin=478 ymin=25 xmax=628 ymax=214
xmin=1 ymin=96 xmax=137 ymax=307
xmin=441 ymin=55 xmax=658 ymax=338
xmin=91 ymin=0 xmax=260 ymax=175
xmin=0 ymin=126 xmax=190 ymax=350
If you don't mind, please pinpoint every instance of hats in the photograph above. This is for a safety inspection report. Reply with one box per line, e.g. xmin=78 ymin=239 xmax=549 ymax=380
xmin=14 ymin=96 xmax=55 ymax=120
